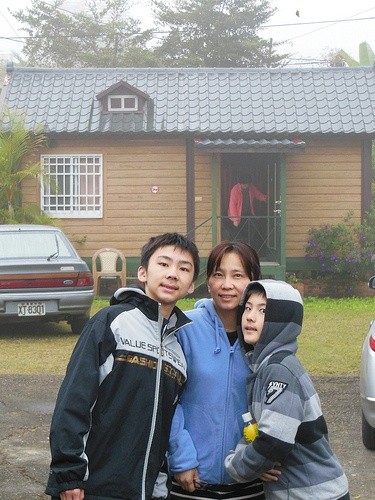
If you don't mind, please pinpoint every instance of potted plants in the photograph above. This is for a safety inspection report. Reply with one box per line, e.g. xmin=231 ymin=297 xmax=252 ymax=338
xmin=288 ymin=273 xmax=305 ymax=296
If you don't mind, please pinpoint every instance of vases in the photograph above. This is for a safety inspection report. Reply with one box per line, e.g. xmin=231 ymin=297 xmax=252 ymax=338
xmin=305 ymin=281 xmax=325 ymax=296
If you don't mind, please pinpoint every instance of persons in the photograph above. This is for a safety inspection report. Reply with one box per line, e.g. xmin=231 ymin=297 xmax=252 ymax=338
xmin=164 ymin=241 xmax=267 ymax=500
xmin=227 ymin=173 xmax=267 ymax=245
xmin=224 ymin=279 xmax=350 ymax=500
xmin=44 ymin=232 xmax=200 ymax=500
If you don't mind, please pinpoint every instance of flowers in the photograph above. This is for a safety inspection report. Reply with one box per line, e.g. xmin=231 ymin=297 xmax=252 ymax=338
xmin=303 ymin=223 xmax=325 ymax=280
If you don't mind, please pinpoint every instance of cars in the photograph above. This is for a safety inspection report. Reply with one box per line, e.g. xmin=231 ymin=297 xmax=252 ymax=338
xmin=0 ymin=225 xmax=95 ymax=334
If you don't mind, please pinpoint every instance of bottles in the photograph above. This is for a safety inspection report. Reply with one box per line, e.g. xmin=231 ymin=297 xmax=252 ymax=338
xmin=241 ymin=412 xmax=260 ymax=445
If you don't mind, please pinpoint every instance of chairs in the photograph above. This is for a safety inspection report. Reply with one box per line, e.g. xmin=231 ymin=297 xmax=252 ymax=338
xmin=92 ymin=247 xmax=126 ymax=296
